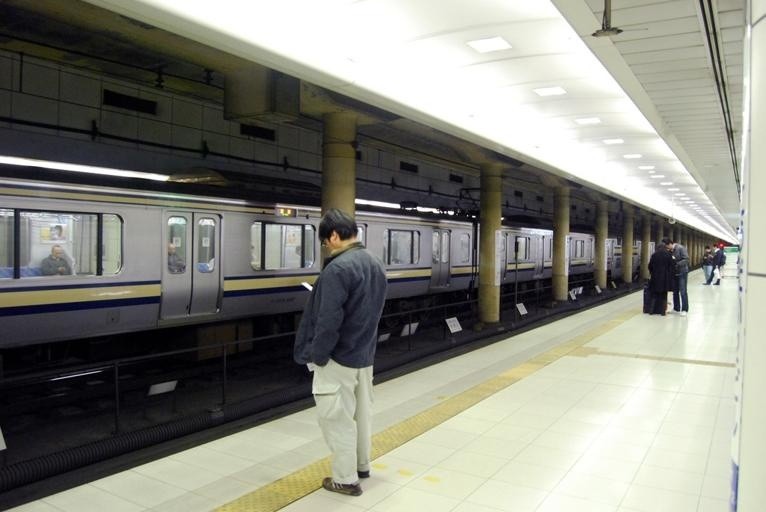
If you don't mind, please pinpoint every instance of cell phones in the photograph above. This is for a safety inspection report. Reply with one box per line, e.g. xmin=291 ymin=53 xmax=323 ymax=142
xmin=300 ymin=280 xmax=313 ymax=293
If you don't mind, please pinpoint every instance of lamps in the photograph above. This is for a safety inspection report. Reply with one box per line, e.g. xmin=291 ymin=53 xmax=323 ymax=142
xmin=591 ymin=0 xmax=622 ymax=37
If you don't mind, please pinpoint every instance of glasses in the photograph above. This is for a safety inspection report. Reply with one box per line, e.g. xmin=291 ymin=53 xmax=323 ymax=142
xmin=321 ymin=236 xmax=328 ymax=248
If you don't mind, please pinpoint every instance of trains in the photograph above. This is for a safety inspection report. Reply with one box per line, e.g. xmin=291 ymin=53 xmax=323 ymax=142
xmin=0 ymin=173 xmax=658 ymax=425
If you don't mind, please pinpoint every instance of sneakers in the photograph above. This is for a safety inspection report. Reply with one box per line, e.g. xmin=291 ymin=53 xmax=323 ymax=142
xmin=323 ymin=470 xmax=369 ymax=495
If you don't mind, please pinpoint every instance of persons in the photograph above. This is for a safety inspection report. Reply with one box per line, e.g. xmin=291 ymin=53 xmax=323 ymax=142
xmin=167 ymin=242 xmax=185 ymax=273
xmin=293 ymin=208 xmax=388 ymax=496
xmin=41 ymin=245 xmax=71 ymax=275
xmin=661 ymin=238 xmax=689 ymax=316
xmin=702 ymin=246 xmax=714 ymax=282
xmin=648 ymin=242 xmax=673 ymax=315
xmin=703 ymin=244 xmax=723 ymax=285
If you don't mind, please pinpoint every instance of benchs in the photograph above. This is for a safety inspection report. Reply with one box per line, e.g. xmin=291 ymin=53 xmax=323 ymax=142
xmin=0 ymin=266 xmax=43 ymax=278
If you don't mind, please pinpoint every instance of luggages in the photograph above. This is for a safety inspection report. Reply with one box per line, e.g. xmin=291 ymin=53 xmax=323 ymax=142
xmin=644 ymin=283 xmax=667 ymax=313
